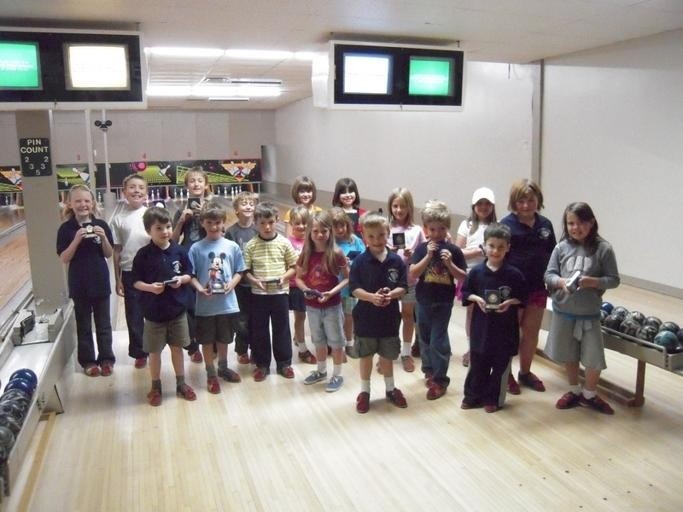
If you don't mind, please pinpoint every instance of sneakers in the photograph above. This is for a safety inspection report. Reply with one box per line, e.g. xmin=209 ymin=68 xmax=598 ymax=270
xmin=325 ymin=376 xmax=344 ymax=392
xmin=277 ymin=365 xmax=294 ymax=379
xmin=207 ymin=376 xmax=221 ymax=394
xmin=177 ymin=384 xmax=196 ymax=401
xmin=556 ymin=391 xmax=580 ymax=410
xmin=149 ymin=388 xmax=161 ymax=406
xmin=404 ymin=341 xmax=546 ymax=414
xmin=252 ymin=366 xmax=271 ymax=382
xmin=385 ymin=388 xmax=407 ymax=409
xmin=303 ymin=370 xmax=327 ymax=385
xmin=237 ymin=345 xmax=359 ymax=364
xmin=84 ymin=348 xmax=204 ymax=376
xmin=356 ymin=392 xmax=370 ymax=413
xmin=580 ymin=390 xmax=615 ymax=415
xmin=218 ymin=368 xmax=240 ymax=383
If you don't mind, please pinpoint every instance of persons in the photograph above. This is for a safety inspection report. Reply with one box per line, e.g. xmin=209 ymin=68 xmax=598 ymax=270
xmin=460 ymin=222 xmax=527 ymax=412
xmin=225 ymin=190 xmax=259 ymax=366
xmin=108 ymin=171 xmax=150 ymax=369
xmin=128 ymin=205 xmax=196 ymax=406
xmin=541 ymin=200 xmax=620 ymax=414
xmin=454 ymin=186 xmax=500 ymax=367
xmin=500 ymin=180 xmax=557 ymax=395
xmin=186 ymin=201 xmax=242 ymax=394
xmin=171 ymin=167 xmax=218 ymax=364
xmin=242 ymin=201 xmax=298 ymax=382
xmin=55 ymin=184 xmax=117 ymax=376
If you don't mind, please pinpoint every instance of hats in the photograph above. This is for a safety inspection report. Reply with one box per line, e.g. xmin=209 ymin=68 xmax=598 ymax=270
xmin=472 ymin=186 xmax=496 ymax=206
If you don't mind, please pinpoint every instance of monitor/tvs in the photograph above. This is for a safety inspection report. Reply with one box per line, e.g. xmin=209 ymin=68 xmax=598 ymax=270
xmin=0 ymin=31 xmax=54 ymax=102
xmin=402 ymin=48 xmax=464 ymax=105
xmin=52 ymin=32 xmax=142 ymax=101
xmin=334 ymin=44 xmax=402 ymax=103
